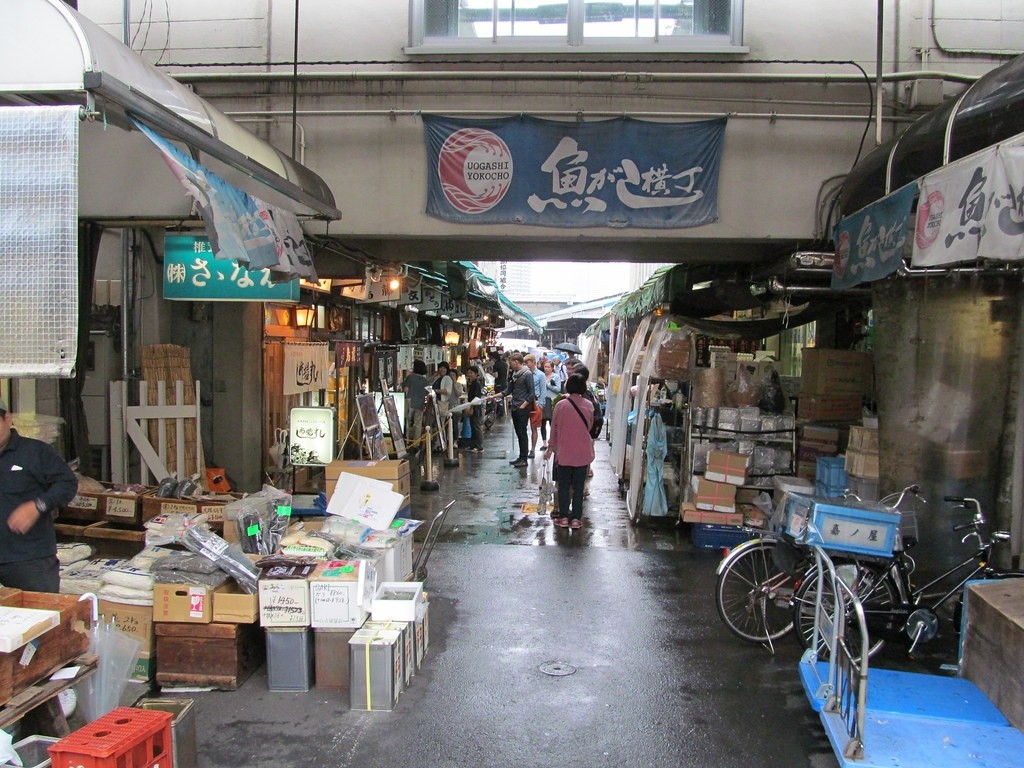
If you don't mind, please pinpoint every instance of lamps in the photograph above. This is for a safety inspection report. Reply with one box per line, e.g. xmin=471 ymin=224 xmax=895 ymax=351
xmin=368 ymin=265 xmax=384 ymax=283
xmin=295 ymin=304 xmax=316 ymax=330
xmin=444 ymin=329 xmax=460 ymax=345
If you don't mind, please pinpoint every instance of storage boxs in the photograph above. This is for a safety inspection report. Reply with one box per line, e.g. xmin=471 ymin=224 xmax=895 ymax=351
xmin=796 ymin=460 xmax=815 ymax=482
xmin=0 ymin=457 xmax=430 ymax=768
xmin=738 ymin=361 xmax=759 ymax=375
xmin=845 ymin=474 xmax=879 ymax=499
xmin=799 ymin=392 xmax=863 ymax=419
xmin=801 ymin=347 xmax=873 ymax=396
xmin=759 ymin=361 xmax=783 ymax=375
xmin=960 ymin=578 xmax=1024 ymax=733
xmin=848 ymin=425 xmax=879 ymax=454
xmin=786 ymin=493 xmax=902 ymax=558
xmin=844 ymin=448 xmax=880 ymax=478
xmin=815 ymin=479 xmax=845 ymax=499
xmin=798 ymin=424 xmax=839 ymax=467
xmin=691 ymin=474 xmax=738 ymax=511
xmin=723 ymin=373 xmax=736 ymax=387
xmin=711 ymin=361 xmax=737 ymax=371
xmin=710 ymin=351 xmax=737 ymax=362
xmin=705 ymin=449 xmax=752 ymax=487
xmin=815 ymin=454 xmax=845 ymax=486
xmin=681 ymin=500 xmax=745 ymax=527
xmin=774 ymin=473 xmax=814 ymax=504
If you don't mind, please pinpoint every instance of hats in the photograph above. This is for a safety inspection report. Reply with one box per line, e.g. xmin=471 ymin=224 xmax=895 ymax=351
xmin=523 ymin=353 xmax=535 ymax=362
xmin=0 ymin=401 xmax=7 ymax=411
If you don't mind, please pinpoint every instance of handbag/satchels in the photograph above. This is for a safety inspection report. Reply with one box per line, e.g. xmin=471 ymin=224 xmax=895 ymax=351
xmin=543 ymin=397 xmax=552 ymax=420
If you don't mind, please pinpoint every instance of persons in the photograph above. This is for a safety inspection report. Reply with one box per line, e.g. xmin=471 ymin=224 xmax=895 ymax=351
xmin=0 ymin=400 xmax=79 ymax=738
xmin=543 ymin=374 xmax=594 ymax=528
xmin=464 ymin=366 xmax=484 ymax=453
xmin=485 ymin=353 xmax=535 ymax=467
xmin=504 ymin=349 xmax=529 ymax=379
xmin=425 ymin=360 xmax=453 ymax=450
xmin=404 ymin=360 xmax=431 ymax=454
xmin=523 ymin=352 xmax=595 ymax=516
xmin=485 ymin=352 xmax=507 ymax=417
xmin=444 ymin=368 xmax=462 ymax=448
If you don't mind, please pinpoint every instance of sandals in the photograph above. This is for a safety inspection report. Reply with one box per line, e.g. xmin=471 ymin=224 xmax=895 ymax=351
xmin=555 ymin=517 xmax=569 ymax=528
xmin=572 ymin=519 xmax=583 ymax=528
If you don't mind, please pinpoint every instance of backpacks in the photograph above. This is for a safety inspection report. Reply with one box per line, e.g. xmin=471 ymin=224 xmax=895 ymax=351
xmin=581 ymin=389 xmax=604 ymax=439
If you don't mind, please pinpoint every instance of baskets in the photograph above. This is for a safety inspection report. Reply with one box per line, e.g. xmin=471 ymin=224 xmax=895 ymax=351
xmin=898 ymin=502 xmax=919 ymax=542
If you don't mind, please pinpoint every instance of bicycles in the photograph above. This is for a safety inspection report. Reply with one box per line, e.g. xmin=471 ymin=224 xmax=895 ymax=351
xmin=713 ymin=483 xmax=1024 ymax=666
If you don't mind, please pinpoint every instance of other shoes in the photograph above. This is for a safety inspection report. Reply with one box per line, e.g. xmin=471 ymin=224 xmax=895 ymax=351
xmin=515 ymin=460 xmax=528 ymax=467
xmin=529 ymin=451 xmax=534 ymax=457
xmin=540 ymin=446 xmax=547 ymax=450
xmin=588 ymin=469 xmax=593 ymax=476
xmin=550 ymin=510 xmax=560 ymax=518
xmin=471 ymin=448 xmax=484 ymax=452
xmin=465 ymin=447 xmax=471 ymax=451
xmin=509 ymin=459 xmax=520 ymax=464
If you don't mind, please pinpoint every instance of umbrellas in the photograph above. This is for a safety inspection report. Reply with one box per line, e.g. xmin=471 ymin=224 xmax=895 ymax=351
xmin=553 ymin=342 xmax=582 ymax=355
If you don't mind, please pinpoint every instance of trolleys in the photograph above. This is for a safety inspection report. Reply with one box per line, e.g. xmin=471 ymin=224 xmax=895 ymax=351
xmin=408 ymin=499 xmax=457 ymax=583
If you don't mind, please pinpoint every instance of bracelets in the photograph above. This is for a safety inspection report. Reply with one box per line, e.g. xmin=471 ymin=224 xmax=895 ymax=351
xmin=546 ymin=448 xmax=551 ymax=454
xmin=35 ymin=496 xmax=47 ymax=514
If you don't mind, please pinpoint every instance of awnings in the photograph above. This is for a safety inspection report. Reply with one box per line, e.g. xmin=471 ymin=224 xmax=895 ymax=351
xmin=404 ymin=261 xmax=543 ymax=335
